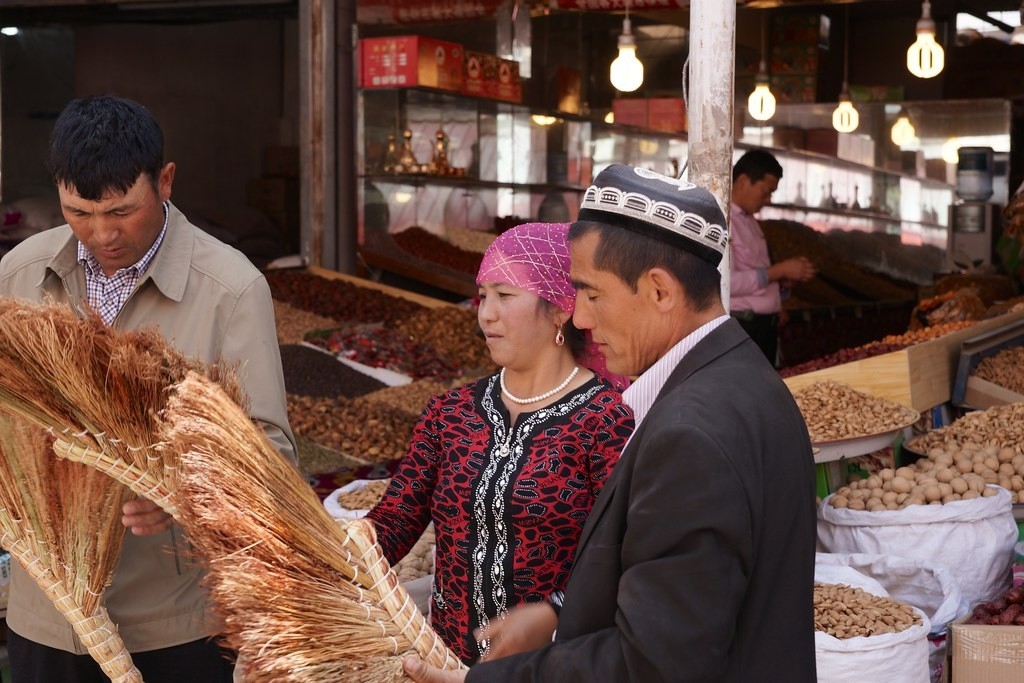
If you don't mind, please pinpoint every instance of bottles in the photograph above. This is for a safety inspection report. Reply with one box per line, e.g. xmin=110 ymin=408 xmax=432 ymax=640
xmin=956 ymin=146 xmax=994 ymax=203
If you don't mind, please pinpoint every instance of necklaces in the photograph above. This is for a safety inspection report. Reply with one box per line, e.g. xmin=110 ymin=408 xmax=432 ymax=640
xmin=500 ymin=366 xmax=578 ymax=405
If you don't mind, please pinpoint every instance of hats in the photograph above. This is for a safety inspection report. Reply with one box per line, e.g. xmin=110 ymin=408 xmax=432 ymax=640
xmin=577 ymin=165 xmax=728 ymax=268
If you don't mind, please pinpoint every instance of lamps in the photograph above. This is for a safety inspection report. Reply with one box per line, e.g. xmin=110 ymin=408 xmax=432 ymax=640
xmin=891 ymin=106 xmax=915 ymax=148
xmin=907 ymin=0 xmax=945 ymax=78
xmin=610 ymin=0 xmax=644 ymax=92
xmin=748 ymin=9 xmax=775 ymax=121
xmin=832 ymin=4 xmax=859 ymax=133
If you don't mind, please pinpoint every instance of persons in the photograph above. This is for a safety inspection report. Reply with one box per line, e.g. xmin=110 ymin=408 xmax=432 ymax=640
xmin=360 ymin=223 xmax=636 ymax=682
xmin=403 ymin=166 xmax=818 ymax=683
xmin=728 ymin=148 xmax=815 ymax=371
xmin=0 ymin=95 xmax=300 ymax=683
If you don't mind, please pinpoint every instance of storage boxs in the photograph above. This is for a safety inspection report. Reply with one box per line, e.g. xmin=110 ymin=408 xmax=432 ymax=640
xmin=941 ymin=614 xmax=1024 ymax=683
xmin=363 ymin=35 xmax=464 ymax=91
xmin=464 ymin=49 xmax=522 ymax=104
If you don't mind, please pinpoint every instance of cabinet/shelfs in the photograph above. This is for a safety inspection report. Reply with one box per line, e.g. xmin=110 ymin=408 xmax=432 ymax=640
xmin=356 ymin=85 xmax=953 ymax=250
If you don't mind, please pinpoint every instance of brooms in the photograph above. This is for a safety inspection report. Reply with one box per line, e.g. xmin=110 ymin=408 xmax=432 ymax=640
xmin=0 ymin=286 xmax=253 ymax=683
xmin=151 ymin=365 xmax=472 ymax=683
